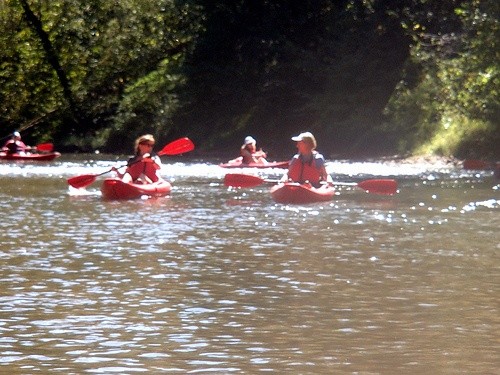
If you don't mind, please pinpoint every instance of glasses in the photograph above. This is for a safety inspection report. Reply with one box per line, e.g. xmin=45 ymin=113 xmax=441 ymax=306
xmin=143 ymin=143 xmax=154 ymax=148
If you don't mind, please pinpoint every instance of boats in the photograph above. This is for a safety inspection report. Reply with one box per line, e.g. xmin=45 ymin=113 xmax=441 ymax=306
xmin=462 ymin=158 xmax=500 ymax=171
xmin=357 ymin=178 xmax=397 ymax=196
xmin=223 ymin=172 xmax=288 ymax=187
xmin=270 ymin=170 xmax=336 ymax=204
xmin=101 ymin=175 xmax=171 ymax=201
xmin=0 ymin=148 xmax=62 ymax=164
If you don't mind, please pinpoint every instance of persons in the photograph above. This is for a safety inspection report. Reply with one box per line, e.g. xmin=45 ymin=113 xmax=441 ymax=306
xmin=2 ymin=131 xmax=31 ymax=155
xmin=112 ymin=134 xmax=161 ymax=184
xmin=286 ymin=132 xmax=328 ymax=189
xmin=241 ymin=136 xmax=268 ymax=164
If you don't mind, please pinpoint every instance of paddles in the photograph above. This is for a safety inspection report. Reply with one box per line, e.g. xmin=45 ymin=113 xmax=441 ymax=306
xmin=223 ymin=173 xmax=396 ymax=195
xmin=67 ymin=138 xmax=195 ymax=192
xmin=0 ymin=144 xmax=52 ymax=153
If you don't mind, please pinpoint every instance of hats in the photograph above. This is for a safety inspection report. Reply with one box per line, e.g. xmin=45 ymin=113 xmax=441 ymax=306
xmin=291 ymin=131 xmax=316 ymax=149
xmin=240 ymin=136 xmax=256 ymax=150
